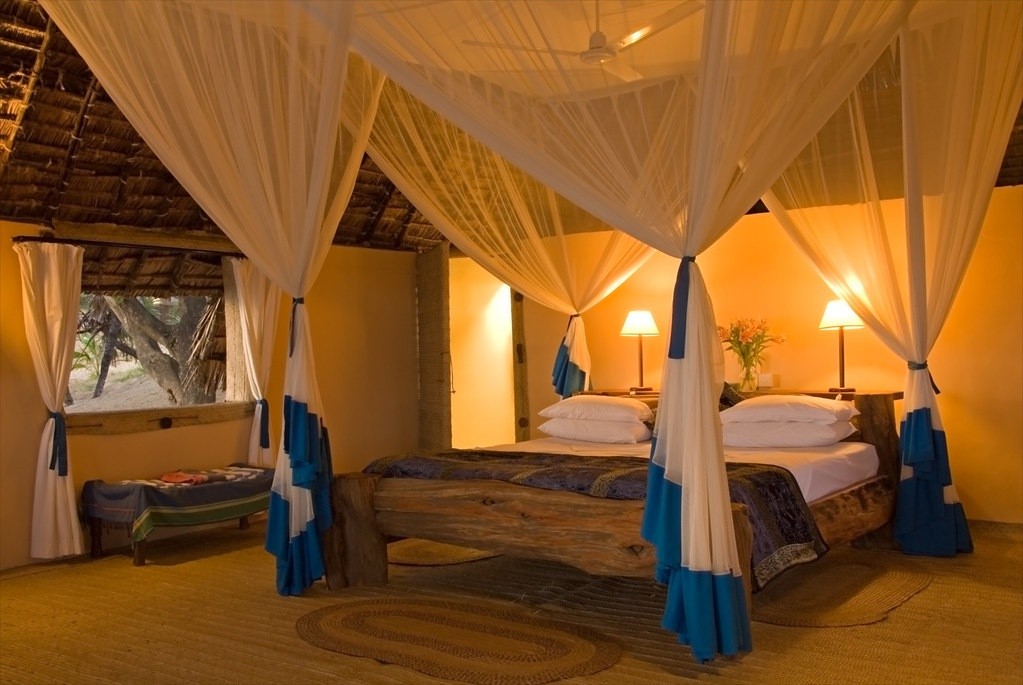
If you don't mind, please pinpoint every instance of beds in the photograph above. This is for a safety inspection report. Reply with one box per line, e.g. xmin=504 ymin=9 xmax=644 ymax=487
xmin=331 ymin=391 xmax=904 ymax=627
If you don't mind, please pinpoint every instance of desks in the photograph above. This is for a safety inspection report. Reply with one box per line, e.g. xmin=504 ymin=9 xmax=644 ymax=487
xmin=82 ymin=460 xmax=275 ymax=567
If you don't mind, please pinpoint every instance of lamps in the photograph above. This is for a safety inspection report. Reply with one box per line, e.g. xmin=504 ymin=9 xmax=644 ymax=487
xmin=619 ymin=310 xmax=659 ymax=391
xmin=818 ymin=299 xmax=865 ymax=394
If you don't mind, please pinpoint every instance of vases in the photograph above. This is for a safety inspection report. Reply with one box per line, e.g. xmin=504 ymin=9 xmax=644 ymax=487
xmin=737 ymin=357 xmax=760 ymax=394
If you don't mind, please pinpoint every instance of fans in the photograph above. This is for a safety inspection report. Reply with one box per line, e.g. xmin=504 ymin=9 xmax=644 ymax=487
xmin=461 ymin=0 xmax=705 ymax=83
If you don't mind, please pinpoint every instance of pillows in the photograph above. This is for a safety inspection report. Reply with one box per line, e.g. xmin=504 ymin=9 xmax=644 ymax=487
xmin=536 ymin=393 xmax=655 ymax=423
xmin=536 ymin=418 xmax=654 ymax=445
xmin=719 ymin=420 xmax=860 ymax=447
xmin=716 ymin=393 xmax=862 ymax=425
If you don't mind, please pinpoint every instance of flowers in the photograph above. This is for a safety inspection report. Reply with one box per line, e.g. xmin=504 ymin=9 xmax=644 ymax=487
xmin=715 ymin=319 xmax=786 ymax=391
